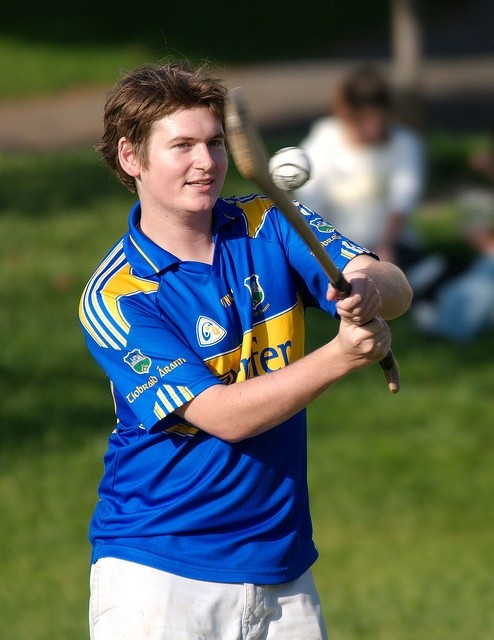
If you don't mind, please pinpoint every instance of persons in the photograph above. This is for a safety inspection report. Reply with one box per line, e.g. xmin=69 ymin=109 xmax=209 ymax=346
xmin=284 ymin=66 xmax=424 ymax=272
xmin=411 ymin=149 xmax=494 ymax=339
xmin=76 ymin=64 xmax=415 ymax=640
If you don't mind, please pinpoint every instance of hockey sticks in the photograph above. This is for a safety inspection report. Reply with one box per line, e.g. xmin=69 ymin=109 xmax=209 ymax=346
xmin=224 ymin=85 xmax=402 ymax=394
